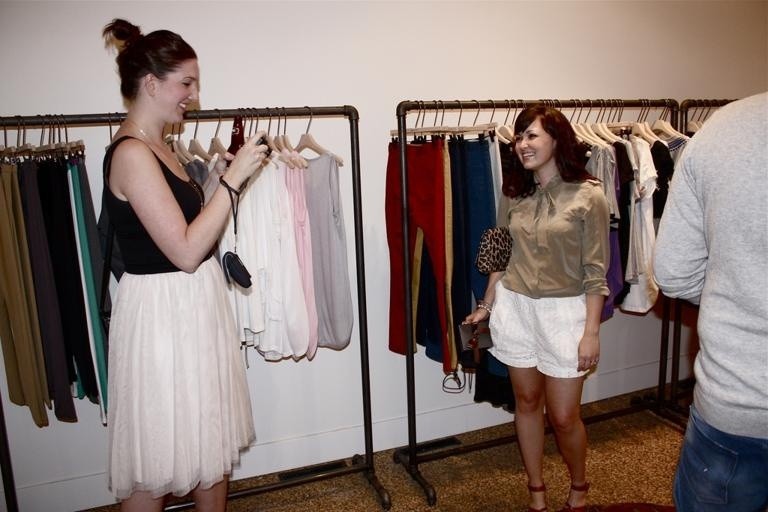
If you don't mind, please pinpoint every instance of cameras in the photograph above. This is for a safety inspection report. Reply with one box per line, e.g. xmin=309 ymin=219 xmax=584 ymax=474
xmin=256 ymin=135 xmax=272 ymax=157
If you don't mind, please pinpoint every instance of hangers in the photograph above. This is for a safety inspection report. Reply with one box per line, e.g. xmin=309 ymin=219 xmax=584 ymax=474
xmin=501 ymin=99 xmax=717 ymax=149
xmin=172 ymin=108 xmax=343 ymax=171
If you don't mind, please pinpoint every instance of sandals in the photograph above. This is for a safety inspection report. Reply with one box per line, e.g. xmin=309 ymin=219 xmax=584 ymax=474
xmin=562 ymin=482 xmax=589 ymax=512
xmin=528 ymin=484 xmax=547 ymax=512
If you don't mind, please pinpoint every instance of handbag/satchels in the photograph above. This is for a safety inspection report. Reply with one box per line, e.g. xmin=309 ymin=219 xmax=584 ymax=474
xmin=223 ymin=252 xmax=251 ymax=288
xmin=474 ymin=226 xmax=512 ymax=275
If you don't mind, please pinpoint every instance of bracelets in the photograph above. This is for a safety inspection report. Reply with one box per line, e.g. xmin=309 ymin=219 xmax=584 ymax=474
xmin=477 ymin=301 xmax=492 ymax=314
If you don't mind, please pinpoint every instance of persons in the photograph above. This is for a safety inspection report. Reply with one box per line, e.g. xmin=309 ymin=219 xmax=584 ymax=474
xmin=651 ymin=90 xmax=768 ymax=510
xmin=461 ymin=102 xmax=610 ymax=510
xmin=101 ymin=16 xmax=267 ymax=511
